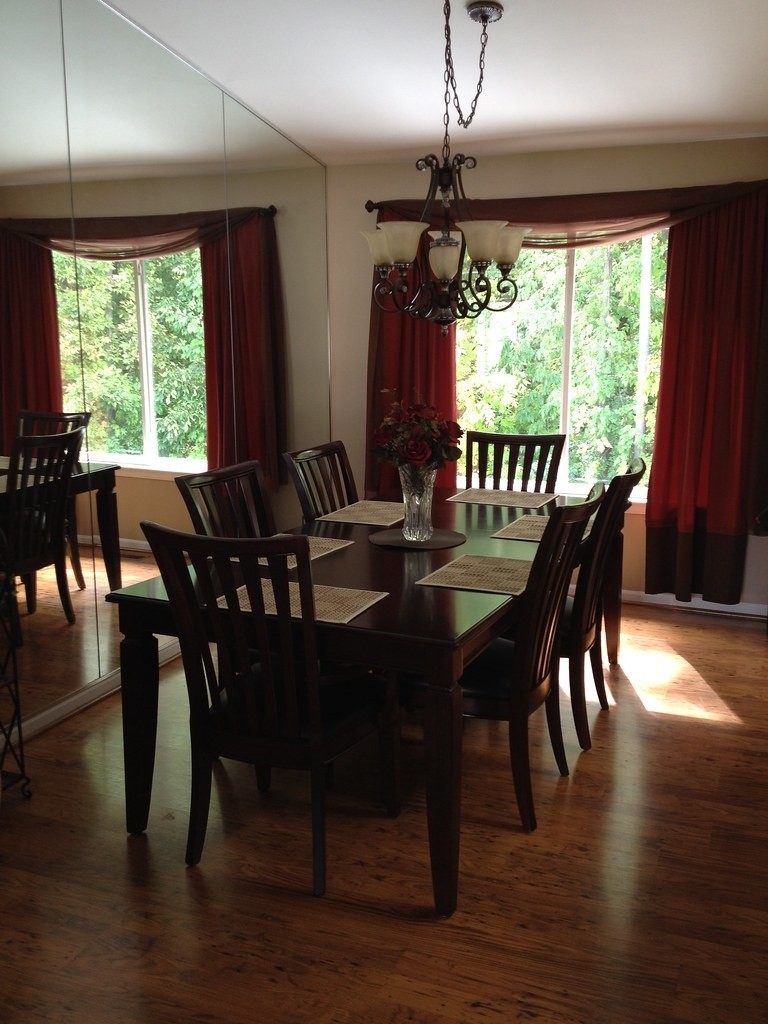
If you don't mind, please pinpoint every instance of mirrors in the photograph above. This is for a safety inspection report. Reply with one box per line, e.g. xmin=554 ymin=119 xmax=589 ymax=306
xmin=1 ymin=0 xmax=328 ymax=750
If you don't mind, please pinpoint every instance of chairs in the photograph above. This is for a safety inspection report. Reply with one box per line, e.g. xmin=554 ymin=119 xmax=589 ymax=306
xmin=381 ymin=482 xmax=605 ymax=834
xmin=465 ymin=429 xmax=567 ymax=497
xmin=136 ymin=519 xmax=402 ymax=902
xmin=0 ymin=424 xmax=86 ymax=647
xmin=282 ymin=438 xmax=358 ymax=524
xmin=16 ymin=410 xmax=91 ymax=593
xmin=174 ymin=459 xmax=276 ymax=538
xmin=513 ymin=455 xmax=648 ymax=755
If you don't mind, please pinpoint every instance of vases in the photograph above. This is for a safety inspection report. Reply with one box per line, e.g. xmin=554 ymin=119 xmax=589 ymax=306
xmin=397 ymin=463 xmax=437 ymax=541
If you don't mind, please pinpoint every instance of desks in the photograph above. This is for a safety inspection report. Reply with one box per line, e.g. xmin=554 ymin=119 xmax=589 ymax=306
xmin=103 ymin=485 xmax=588 ymax=921
xmin=0 ymin=456 xmax=125 ymax=592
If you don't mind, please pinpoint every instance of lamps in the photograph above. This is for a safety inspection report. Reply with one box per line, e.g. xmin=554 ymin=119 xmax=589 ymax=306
xmin=357 ymin=1 xmax=537 ymax=341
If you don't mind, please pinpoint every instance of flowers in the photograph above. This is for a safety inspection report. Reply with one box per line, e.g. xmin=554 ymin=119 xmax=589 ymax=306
xmin=372 ymin=399 xmax=463 ymax=529
